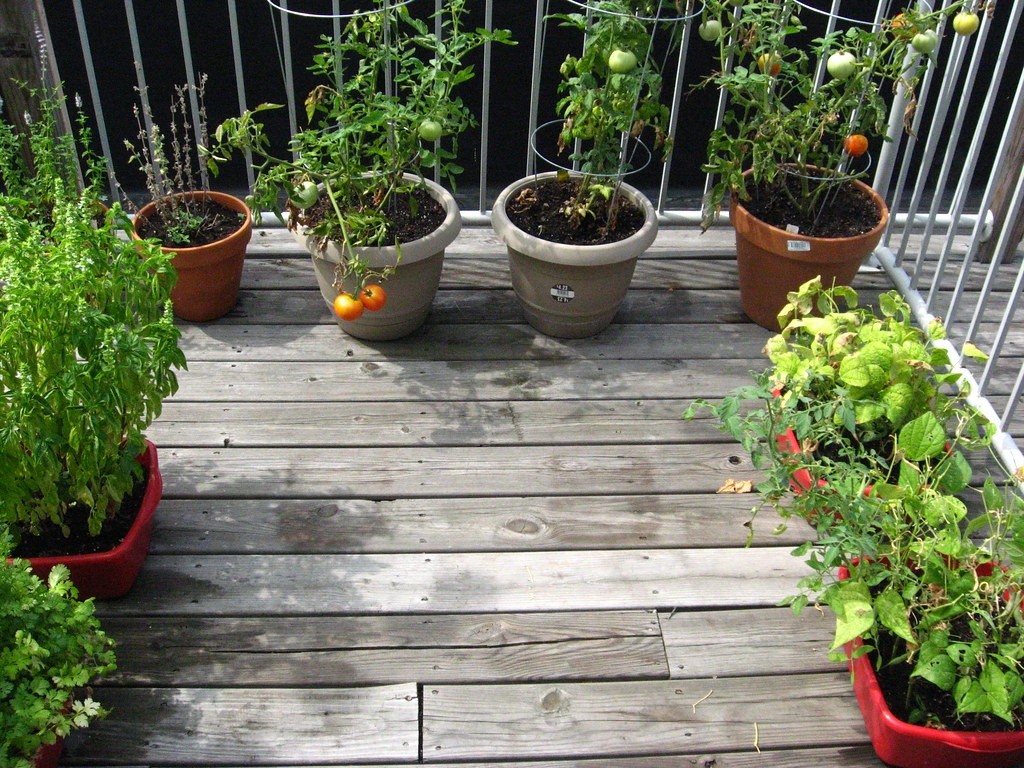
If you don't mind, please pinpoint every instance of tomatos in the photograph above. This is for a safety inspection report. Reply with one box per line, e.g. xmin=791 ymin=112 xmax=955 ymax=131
xmin=419 ymin=119 xmax=442 ymax=141
xmin=699 ymin=0 xmax=979 ymax=154
xmin=334 ymin=285 xmax=386 ymax=321
xmin=289 ymin=180 xmax=318 ymax=210
xmin=608 ymin=48 xmax=637 ymax=74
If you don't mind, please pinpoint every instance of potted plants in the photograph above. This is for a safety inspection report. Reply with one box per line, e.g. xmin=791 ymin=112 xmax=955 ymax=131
xmin=752 ymin=470 xmax=1024 ymax=768
xmin=491 ymin=0 xmax=705 ymax=338
xmin=0 ymin=527 xmax=119 ymax=768
xmin=680 ymin=271 xmax=991 ymax=556
xmin=193 ymin=0 xmax=519 ymax=343
xmin=0 ymin=174 xmax=207 ymax=599
xmin=106 ymin=73 xmax=252 ymax=322
xmin=0 ymin=21 xmax=118 ymax=254
xmin=694 ymin=0 xmax=996 ymax=330
